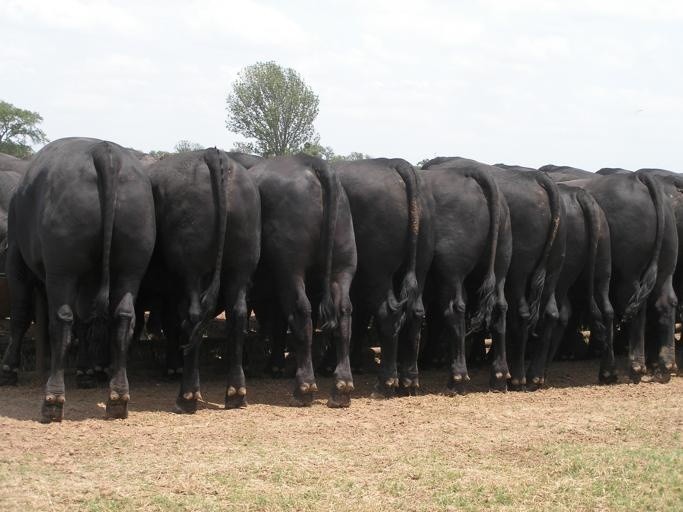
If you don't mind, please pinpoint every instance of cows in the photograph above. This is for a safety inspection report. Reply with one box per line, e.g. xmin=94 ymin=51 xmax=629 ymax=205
xmin=0 ymin=137 xmax=683 ymax=424
